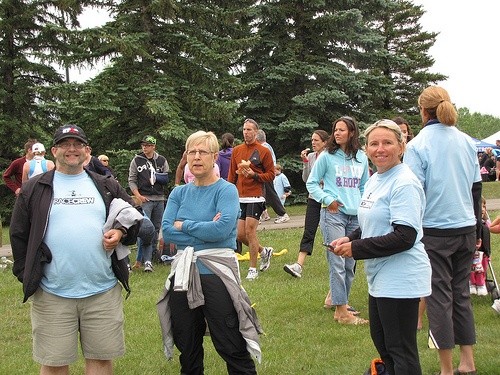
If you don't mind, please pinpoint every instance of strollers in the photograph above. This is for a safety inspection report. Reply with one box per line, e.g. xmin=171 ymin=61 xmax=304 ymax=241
xmin=472 ymin=222 xmax=500 ymax=301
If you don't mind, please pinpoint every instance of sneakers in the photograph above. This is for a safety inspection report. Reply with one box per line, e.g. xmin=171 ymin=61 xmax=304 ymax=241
xmin=259 ymin=247 xmax=274 ymax=272
xmin=283 ymin=263 xmax=303 ymax=278
xmin=246 ymin=266 xmax=258 ymax=281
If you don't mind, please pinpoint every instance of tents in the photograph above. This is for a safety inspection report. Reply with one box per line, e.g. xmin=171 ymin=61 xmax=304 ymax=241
xmin=471 ymin=135 xmax=494 ymax=153
xmin=482 ymin=131 xmax=500 ymax=143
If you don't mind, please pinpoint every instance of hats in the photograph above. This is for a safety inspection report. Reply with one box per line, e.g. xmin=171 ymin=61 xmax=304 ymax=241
xmin=32 ymin=143 xmax=45 ymax=153
xmin=53 ymin=125 xmax=88 ymax=146
xmin=138 ymin=135 xmax=156 ymax=144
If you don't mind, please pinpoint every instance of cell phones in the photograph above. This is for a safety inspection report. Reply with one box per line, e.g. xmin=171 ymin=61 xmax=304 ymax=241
xmin=321 ymin=242 xmax=334 ymax=250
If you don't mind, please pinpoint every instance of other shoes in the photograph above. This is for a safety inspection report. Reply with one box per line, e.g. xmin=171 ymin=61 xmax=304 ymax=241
xmin=274 ymin=213 xmax=290 ymax=224
xmin=144 ymin=261 xmax=156 ymax=273
xmin=470 ymin=285 xmax=477 ymax=295
xmin=476 ymin=285 xmax=488 ymax=296
xmin=128 ymin=264 xmax=132 ymax=272
xmin=260 ymin=209 xmax=270 ymax=221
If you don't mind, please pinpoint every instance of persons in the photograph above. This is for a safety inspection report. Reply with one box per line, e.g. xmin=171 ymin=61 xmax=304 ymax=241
xmin=402 ymin=86 xmax=483 ymax=375
xmin=3 ymin=117 xmax=500 ymax=375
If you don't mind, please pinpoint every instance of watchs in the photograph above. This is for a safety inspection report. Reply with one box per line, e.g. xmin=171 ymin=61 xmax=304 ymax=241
xmin=253 ymin=173 xmax=258 ymax=179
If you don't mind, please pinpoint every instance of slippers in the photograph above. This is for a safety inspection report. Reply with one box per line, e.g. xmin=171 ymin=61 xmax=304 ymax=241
xmin=434 ymin=367 xmax=478 ymax=375
xmin=333 ymin=311 xmax=369 ymax=325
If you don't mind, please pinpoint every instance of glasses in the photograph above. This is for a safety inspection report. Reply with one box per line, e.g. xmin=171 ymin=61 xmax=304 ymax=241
xmin=186 ymin=150 xmax=215 ymax=155
xmin=101 ymin=159 xmax=108 ymax=162
xmin=57 ymin=141 xmax=85 ymax=148
xmin=340 ymin=116 xmax=352 ymax=123
xmin=141 ymin=143 xmax=153 ymax=146
xmin=243 ymin=118 xmax=254 ymax=123
